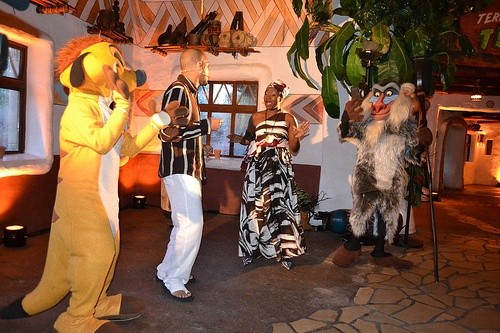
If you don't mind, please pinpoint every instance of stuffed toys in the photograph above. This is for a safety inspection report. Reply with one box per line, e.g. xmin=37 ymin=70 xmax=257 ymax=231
xmin=0 ymin=34 xmax=189 ymax=333
xmin=332 ymin=80 xmax=433 ymax=269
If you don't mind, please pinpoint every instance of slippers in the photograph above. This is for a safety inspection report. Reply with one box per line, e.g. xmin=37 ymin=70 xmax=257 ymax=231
xmin=165 ymin=282 xmax=194 ymax=302
xmin=156 ymin=273 xmax=195 ymax=281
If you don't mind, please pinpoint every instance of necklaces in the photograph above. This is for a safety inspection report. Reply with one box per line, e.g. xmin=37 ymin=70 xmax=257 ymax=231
xmin=265 ymin=109 xmax=280 ymax=142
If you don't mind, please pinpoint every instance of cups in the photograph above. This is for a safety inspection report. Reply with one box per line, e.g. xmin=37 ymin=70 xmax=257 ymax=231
xmin=214 ymin=149 xmax=221 ymax=159
xmin=219 ymin=120 xmax=224 ymax=129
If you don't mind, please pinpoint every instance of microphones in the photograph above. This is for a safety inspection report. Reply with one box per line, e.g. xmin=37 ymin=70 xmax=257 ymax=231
xmin=202 ymin=73 xmax=209 ymax=78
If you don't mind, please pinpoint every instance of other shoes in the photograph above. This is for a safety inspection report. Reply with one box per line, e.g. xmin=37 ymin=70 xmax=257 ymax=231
xmin=242 ymin=257 xmax=254 ymax=266
xmin=282 ymin=261 xmax=292 ymax=270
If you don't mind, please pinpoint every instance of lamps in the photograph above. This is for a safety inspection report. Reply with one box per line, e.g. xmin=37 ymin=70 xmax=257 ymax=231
xmin=132 ymin=195 xmax=147 ymax=209
xmin=3 ymin=224 xmax=28 ymax=249
xmin=470 ymin=87 xmax=484 ymax=101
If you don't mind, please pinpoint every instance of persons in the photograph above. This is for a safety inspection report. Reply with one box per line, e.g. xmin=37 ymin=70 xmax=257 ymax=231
xmin=155 ymin=49 xmax=223 ymax=302
xmin=226 ymin=79 xmax=310 ymax=270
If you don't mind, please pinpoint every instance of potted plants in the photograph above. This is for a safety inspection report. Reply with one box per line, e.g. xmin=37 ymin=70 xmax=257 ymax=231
xmin=293 ymin=181 xmax=312 ymax=230
